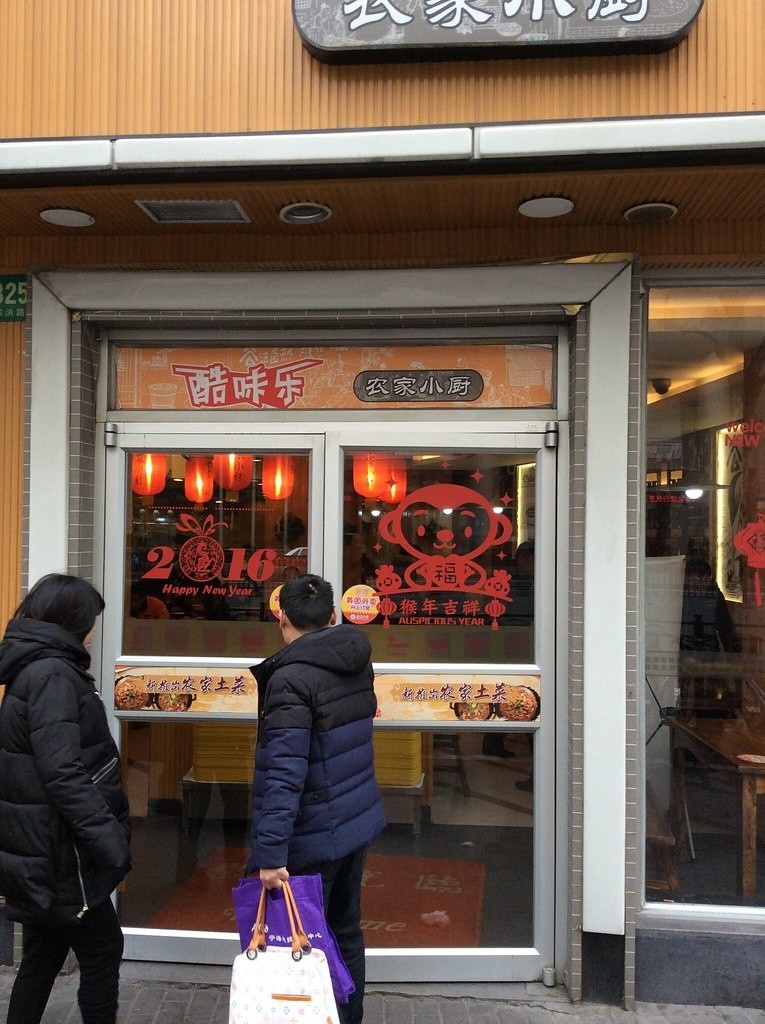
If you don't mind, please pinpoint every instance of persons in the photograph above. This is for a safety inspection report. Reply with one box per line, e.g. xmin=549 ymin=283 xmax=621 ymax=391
xmin=130 ymin=580 xmax=169 ymax=620
xmin=360 ymin=545 xmax=423 ymax=589
xmin=680 ymin=559 xmax=740 ymax=680
xmin=0 ymin=573 xmax=134 ymax=1024
xmin=343 ymin=523 xmax=377 ymax=590
xmin=481 ymin=540 xmax=534 ymax=759
xmin=243 ymin=573 xmax=388 ymax=1024
xmin=254 ymin=512 xmax=305 ymax=621
xmin=172 ymin=764 xmax=249 ymax=886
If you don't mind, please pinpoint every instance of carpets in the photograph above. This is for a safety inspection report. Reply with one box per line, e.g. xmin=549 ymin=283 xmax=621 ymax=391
xmin=140 ymin=844 xmax=486 ymax=948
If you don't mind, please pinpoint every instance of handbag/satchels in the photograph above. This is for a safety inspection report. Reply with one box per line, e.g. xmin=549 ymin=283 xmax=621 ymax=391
xmin=228 ymin=879 xmax=339 ymax=1024
xmin=232 ymin=873 xmax=355 ymax=1004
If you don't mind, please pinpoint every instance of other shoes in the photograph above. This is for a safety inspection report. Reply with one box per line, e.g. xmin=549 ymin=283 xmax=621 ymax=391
xmin=515 ymin=774 xmax=533 ymax=791
xmin=480 ymin=743 xmax=516 ymax=758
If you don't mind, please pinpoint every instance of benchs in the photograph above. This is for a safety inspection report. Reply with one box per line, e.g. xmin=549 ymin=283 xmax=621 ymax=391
xmin=434 ymin=732 xmax=472 ymax=797
xmin=646 ymin=780 xmax=682 ymax=902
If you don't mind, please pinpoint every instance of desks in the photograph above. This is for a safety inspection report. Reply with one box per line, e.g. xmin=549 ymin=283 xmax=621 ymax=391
xmin=666 ymin=691 xmax=765 ymax=899
xmin=178 ymin=766 xmax=429 ymax=876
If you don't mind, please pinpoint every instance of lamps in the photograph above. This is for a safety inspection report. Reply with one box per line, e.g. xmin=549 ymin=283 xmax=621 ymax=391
xmin=40 ymin=209 xmax=96 ymax=228
xmin=518 ymin=198 xmax=575 ymax=219
